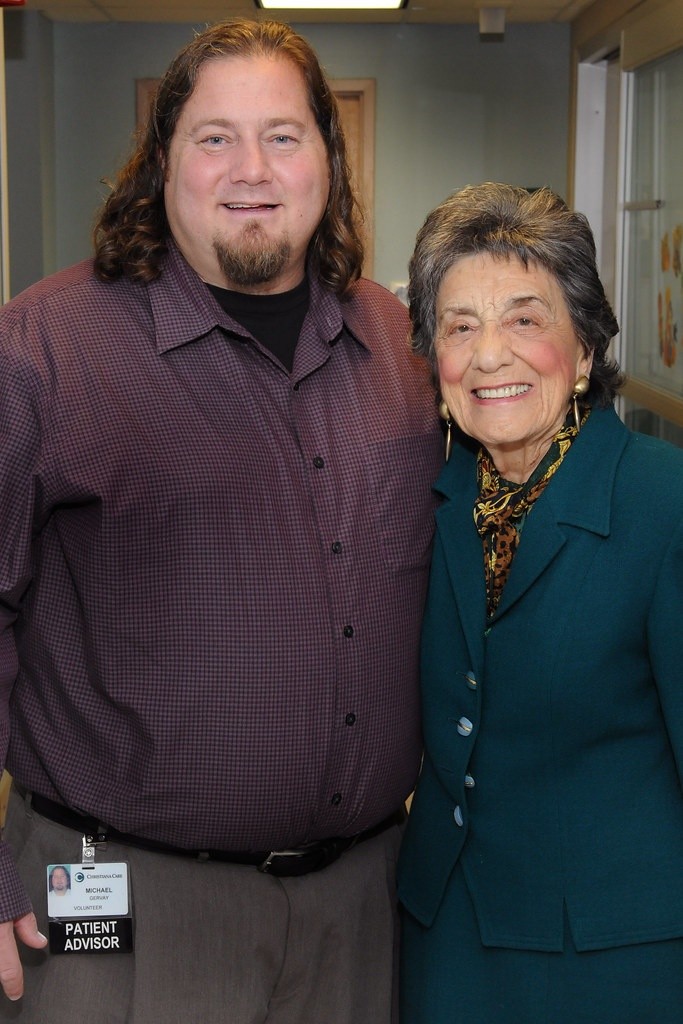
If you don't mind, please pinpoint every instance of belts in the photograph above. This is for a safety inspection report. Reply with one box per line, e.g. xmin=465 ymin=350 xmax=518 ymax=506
xmin=15 ymin=783 xmax=405 ymax=881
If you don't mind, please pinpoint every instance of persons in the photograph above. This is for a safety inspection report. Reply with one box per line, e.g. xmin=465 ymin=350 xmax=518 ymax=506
xmin=393 ymin=182 xmax=683 ymax=1024
xmin=49 ymin=866 xmax=71 ymax=897
xmin=0 ymin=18 xmax=448 ymax=1024
